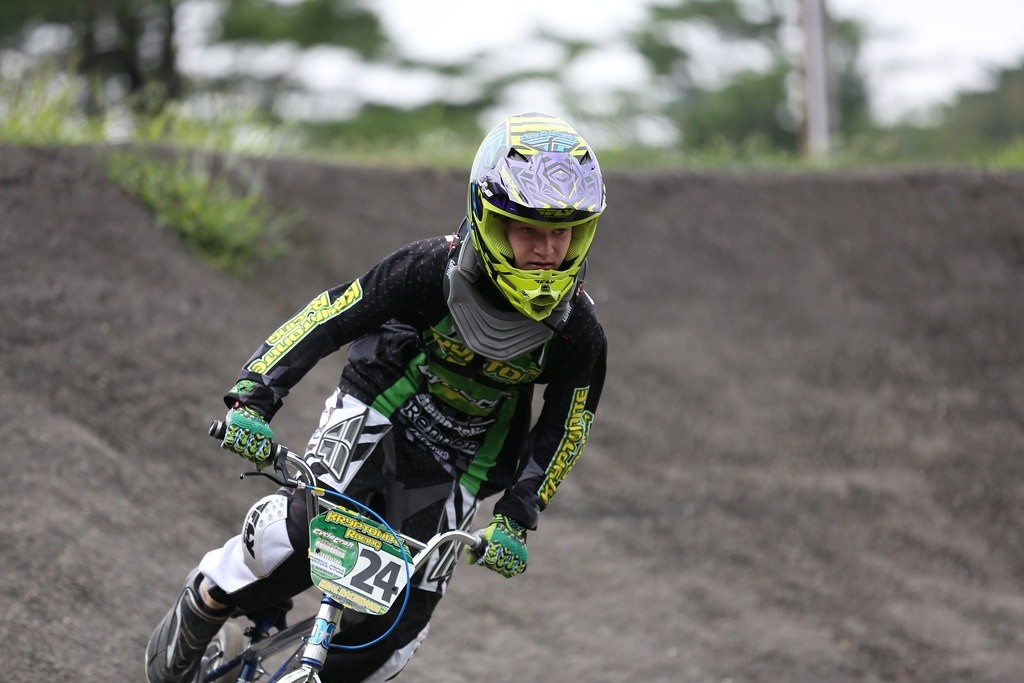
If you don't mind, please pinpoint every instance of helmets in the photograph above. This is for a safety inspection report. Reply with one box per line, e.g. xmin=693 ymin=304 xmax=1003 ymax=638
xmin=466 ymin=114 xmax=607 ymax=322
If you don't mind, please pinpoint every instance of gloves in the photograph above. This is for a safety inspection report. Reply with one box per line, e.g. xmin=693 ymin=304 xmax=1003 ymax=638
xmin=464 ymin=513 xmax=529 ymax=579
xmin=221 ymin=402 xmax=276 ymax=473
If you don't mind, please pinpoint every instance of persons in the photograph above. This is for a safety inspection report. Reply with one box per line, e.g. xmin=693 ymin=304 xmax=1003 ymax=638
xmin=144 ymin=112 xmax=609 ymax=683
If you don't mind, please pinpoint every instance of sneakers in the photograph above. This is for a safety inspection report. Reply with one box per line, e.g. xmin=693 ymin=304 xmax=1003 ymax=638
xmin=146 ymin=565 xmax=229 ymax=683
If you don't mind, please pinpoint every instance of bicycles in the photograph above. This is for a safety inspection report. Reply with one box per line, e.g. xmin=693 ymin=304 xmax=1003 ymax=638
xmin=181 ymin=418 xmax=490 ymax=683
